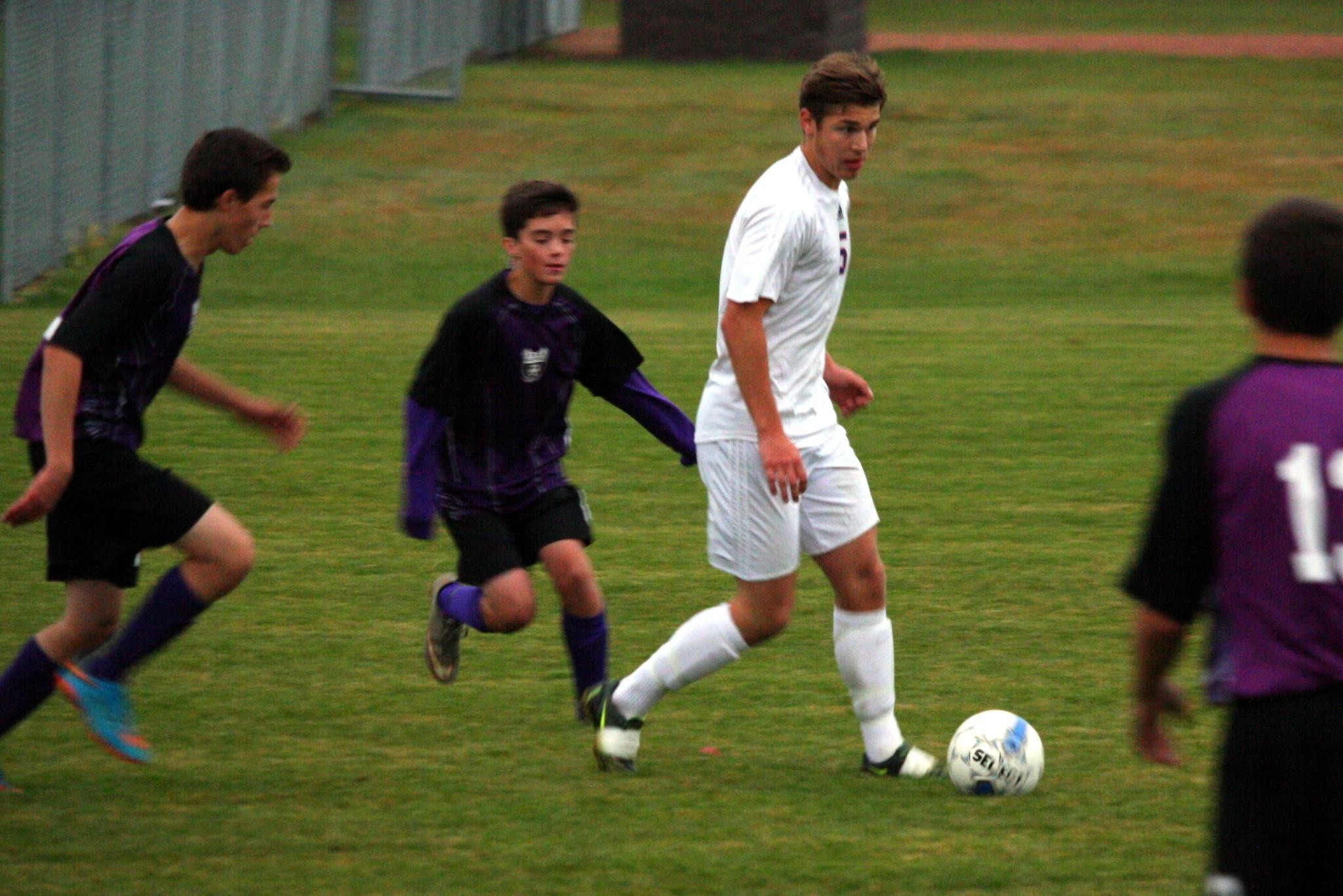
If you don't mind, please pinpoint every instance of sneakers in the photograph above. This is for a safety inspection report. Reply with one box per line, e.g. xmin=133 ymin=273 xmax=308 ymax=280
xmin=584 ymin=676 xmax=646 ymax=777
xmin=860 ymin=740 xmax=949 ymax=777
xmin=424 ymin=571 xmax=463 ymax=684
xmin=53 ymin=657 xmax=153 ymax=766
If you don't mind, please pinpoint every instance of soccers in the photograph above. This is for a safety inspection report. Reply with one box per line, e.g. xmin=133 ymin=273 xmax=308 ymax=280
xmin=945 ymin=706 xmax=1044 ymax=796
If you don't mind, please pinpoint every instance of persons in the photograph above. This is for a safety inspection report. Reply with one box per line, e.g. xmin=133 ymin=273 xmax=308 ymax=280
xmin=578 ymin=51 xmax=946 ymax=782
xmin=400 ymin=180 xmax=699 ymax=723
xmin=1111 ymin=195 xmax=1343 ymax=895
xmin=0 ymin=129 xmax=310 ymax=769
